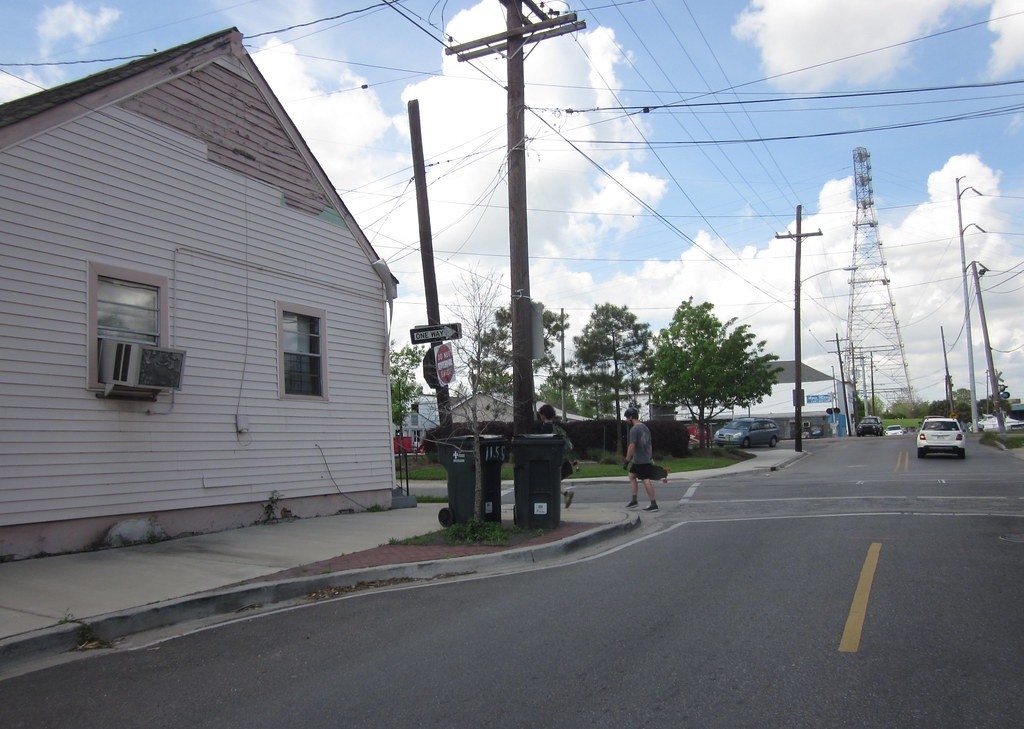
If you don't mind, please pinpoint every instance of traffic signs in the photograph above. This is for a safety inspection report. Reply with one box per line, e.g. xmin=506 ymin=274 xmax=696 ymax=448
xmin=409 ymin=323 xmax=461 ymax=344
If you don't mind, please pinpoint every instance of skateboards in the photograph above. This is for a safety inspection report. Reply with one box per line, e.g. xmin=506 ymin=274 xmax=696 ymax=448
xmin=560 ymin=460 xmax=580 ymax=480
xmin=623 ymin=460 xmax=672 ymax=485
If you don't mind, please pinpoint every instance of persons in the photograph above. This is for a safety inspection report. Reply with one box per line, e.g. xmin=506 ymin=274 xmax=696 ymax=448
xmin=539 ymin=404 xmax=574 ymax=508
xmin=623 ymin=408 xmax=659 ymax=512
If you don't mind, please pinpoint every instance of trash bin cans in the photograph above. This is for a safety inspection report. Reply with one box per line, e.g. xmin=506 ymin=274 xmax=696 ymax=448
xmin=513 ymin=432 xmax=567 ymax=529
xmin=437 ymin=433 xmax=509 ymax=526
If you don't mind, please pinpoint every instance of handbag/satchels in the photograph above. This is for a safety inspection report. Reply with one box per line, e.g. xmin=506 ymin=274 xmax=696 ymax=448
xmin=650 ymin=466 xmax=668 ymax=480
xmin=562 ymin=462 xmax=573 ymax=478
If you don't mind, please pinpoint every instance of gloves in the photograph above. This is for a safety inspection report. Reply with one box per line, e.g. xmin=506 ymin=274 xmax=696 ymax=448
xmin=623 ymin=459 xmax=629 ymax=470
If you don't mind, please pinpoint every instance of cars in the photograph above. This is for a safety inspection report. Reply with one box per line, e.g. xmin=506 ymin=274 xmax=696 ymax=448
xmin=884 ymin=425 xmax=904 ymax=437
xmin=713 ymin=417 xmax=782 ymax=449
xmin=916 ymin=416 xmax=965 ymax=459
xmin=856 ymin=416 xmax=883 ymax=437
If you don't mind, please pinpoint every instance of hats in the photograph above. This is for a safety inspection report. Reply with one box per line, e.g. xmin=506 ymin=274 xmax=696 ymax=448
xmin=539 ymin=405 xmax=555 ymax=419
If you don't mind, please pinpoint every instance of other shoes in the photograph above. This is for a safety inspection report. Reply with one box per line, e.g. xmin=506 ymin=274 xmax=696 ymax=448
xmin=642 ymin=505 xmax=658 ymax=512
xmin=565 ymin=491 xmax=573 ymax=508
xmin=625 ymin=502 xmax=639 ymax=510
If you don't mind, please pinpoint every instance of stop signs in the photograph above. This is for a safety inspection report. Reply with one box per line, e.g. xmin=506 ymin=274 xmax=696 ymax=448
xmin=433 ymin=342 xmax=456 ymax=388
